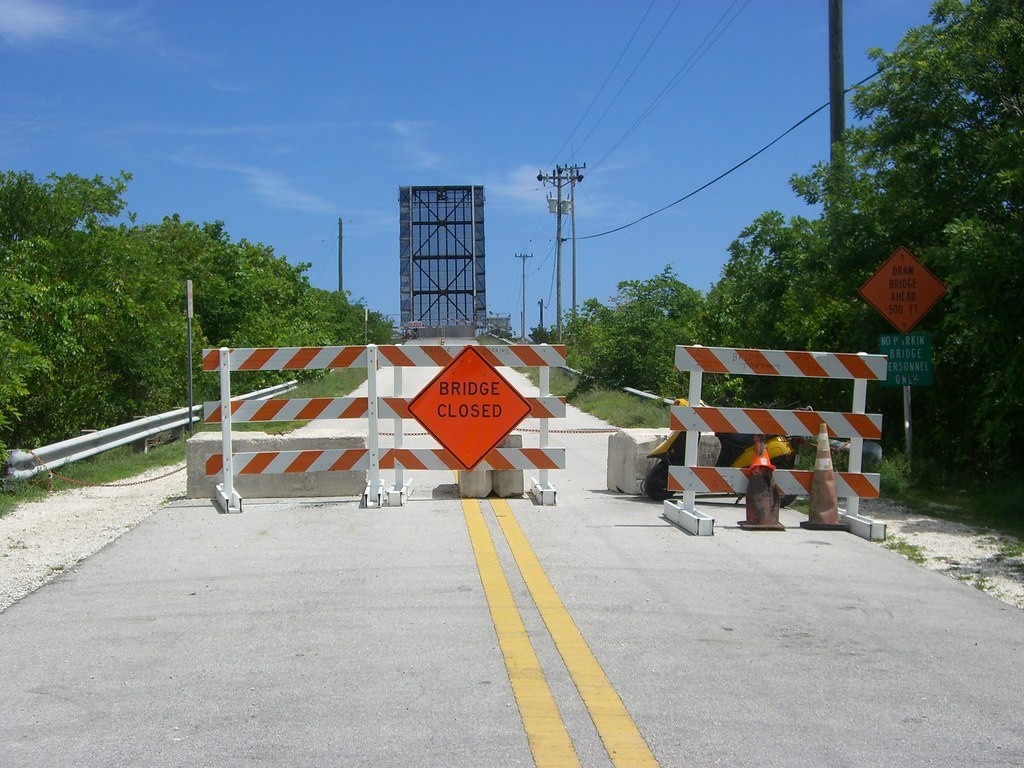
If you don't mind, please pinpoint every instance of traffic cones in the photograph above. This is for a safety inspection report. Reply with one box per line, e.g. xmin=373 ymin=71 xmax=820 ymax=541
xmin=737 ymin=436 xmax=785 ymax=531
xmin=800 ymin=423 xmax=847 ymax=530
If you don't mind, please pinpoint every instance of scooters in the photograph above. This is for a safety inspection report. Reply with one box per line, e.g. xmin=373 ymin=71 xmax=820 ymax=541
xmin=644 ymin=399 xmax=813 ymax=508
